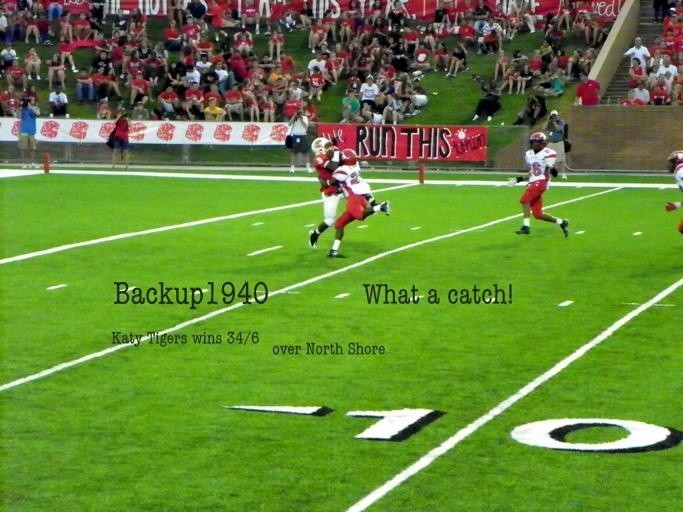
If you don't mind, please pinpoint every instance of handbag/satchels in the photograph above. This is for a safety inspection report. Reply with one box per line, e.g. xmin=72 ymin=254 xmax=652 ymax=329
xmin=285 ymin=135 xmax=292 ymax=148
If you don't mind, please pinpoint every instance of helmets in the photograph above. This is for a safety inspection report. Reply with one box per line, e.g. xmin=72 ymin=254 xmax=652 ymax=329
xmin=310 ymin=137 xmax=329 ymax=154
xmin=529 ymin=132 xmax=546 ymax=149
xmin=666 ymin=151 xmax=682 ymax=173
xmin=339 ymin=149 xmax=356 ymax=165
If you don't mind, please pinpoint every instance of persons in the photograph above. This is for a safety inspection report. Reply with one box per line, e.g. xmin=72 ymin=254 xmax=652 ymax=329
xmin=0 ymin=1 xmax=683 ymax=125
xmin=508 ymin=132 xmax=568 ymax=236
xmin=309 ymin=137 xmax=346 ymax=250
xmin=287 ymin=107 xmax=315 ymax=173
xmin=327 ymin=148 xmax=390 ymax=257
xmin=113 ymin=112 xmax=129 ymax=170
xmin=665 ymin=151 xmax=683 ymax=234
xmin=16 ymin=95 xmax=41 ymax=169
xmin=544 ymin=111 xmax=568 ymax=182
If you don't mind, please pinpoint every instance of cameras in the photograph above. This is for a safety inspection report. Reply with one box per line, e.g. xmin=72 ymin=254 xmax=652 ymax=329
xmin=550 ymin=115 xmax=555 ymax=120
xmin=121 ymin=111 xmax=128 ymax=117
xmin=297 ymin=111 xmax=302 ymax=115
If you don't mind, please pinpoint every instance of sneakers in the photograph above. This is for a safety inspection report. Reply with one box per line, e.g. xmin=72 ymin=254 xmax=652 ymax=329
xmin=560 ymin=219 xmax=567 ymax=237
xmin=515 ymin=226 xmax=529 ymax=234
xmin=308 ymin=200 xmax=390 ymax=257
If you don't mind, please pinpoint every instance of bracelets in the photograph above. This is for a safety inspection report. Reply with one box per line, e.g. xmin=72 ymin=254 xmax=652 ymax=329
xmin=517 ymin=177 xmax=523 ymax=183
xmin=551 ymin=167 xmax=556 ymax=177
xmin=331 ymin=151 xmax=340 ymax=162
xmin=674 ymin=201 xmax=681 ymax=209
xmin=326 ymin=179 xmax=331 ymax=187
